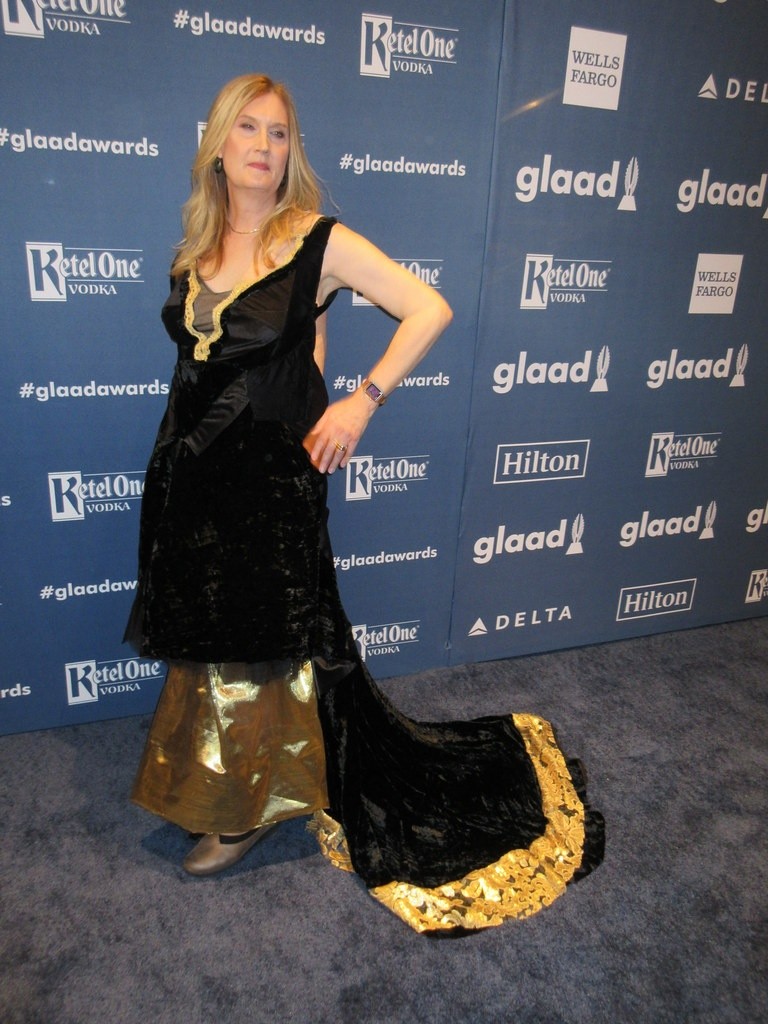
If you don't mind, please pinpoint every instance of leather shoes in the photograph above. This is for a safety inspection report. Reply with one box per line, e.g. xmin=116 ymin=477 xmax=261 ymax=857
xmin=183 ymin=823 xmax=276 ymax=875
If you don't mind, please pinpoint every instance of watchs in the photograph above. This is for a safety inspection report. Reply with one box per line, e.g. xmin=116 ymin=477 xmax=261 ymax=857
xmin=361 ymin=380 xmax=386 ymax=405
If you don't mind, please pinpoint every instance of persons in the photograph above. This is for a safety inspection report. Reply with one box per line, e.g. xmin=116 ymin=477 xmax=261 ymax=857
xmin=120 ymin=73 xmax=609 ymax=934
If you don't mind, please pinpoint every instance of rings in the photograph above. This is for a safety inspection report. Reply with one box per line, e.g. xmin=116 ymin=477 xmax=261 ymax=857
xmin=337 ymin=444 xmax=347 ymax=451
